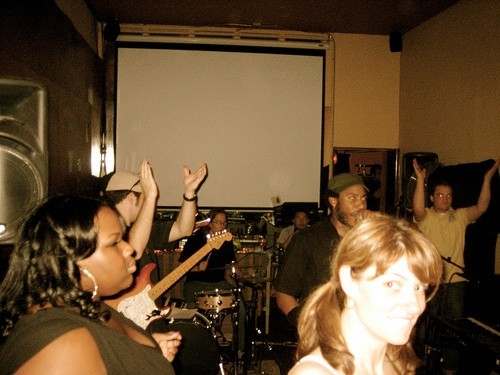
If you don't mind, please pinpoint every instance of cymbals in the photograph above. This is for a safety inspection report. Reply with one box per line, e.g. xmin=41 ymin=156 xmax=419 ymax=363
xmin=212 ymin=263 xmax=262 ymax=270
xmin=243 ymin=276 xmax=268 ymax=283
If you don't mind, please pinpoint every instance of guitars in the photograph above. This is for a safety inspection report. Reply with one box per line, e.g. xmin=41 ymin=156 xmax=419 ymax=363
xmin=104 ymin=228 xmax=233 ymax=330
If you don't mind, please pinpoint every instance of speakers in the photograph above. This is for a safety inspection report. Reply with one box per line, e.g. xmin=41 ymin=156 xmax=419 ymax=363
xmin=0 ymin=77 xmax=49 ymax=247
xmin=389 ymin=30 xmax=402 ymax=52
xmin=104 ymin=19 xmax=121 ymax=42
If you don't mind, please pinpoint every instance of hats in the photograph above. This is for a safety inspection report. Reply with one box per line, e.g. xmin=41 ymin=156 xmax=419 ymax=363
xmin=106 ymin=171 xmax=144 ymax=192
xmin=329 ymin=173 xmax=370 ymax=193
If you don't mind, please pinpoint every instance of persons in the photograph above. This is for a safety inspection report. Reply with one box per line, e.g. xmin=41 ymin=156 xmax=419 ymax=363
xmin=276 ymin=212 xmax=310 ymax=249
xmin=65 ymin=160 xmax=207 ymax=363
xmin=0 ymin=197 xmax=175 ymax=375
xmin=413 ymin=158 xmax=500 ymax=318
xmin=177 ymin=210 xmax=255 ymax=363
xmin=275 ymin=174 xmax=367 ymax=327
xmin=288 ymin=215 xmax=443 ymax=375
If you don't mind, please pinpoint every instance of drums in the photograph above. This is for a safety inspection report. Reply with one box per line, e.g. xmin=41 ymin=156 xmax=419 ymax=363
xmin=194 ymin=289 xmax=236 ymax=314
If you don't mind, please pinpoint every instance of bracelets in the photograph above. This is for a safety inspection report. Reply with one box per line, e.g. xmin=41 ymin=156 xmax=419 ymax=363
xmin=183 ymin=193 xmax=197 ymax=201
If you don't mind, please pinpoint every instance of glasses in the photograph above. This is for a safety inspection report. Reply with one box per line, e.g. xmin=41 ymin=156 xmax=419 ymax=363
xmin=213 ymin=220 xmax=227 ymax=225
xmin=435 ymin=194 xmax=452 ymax=198
